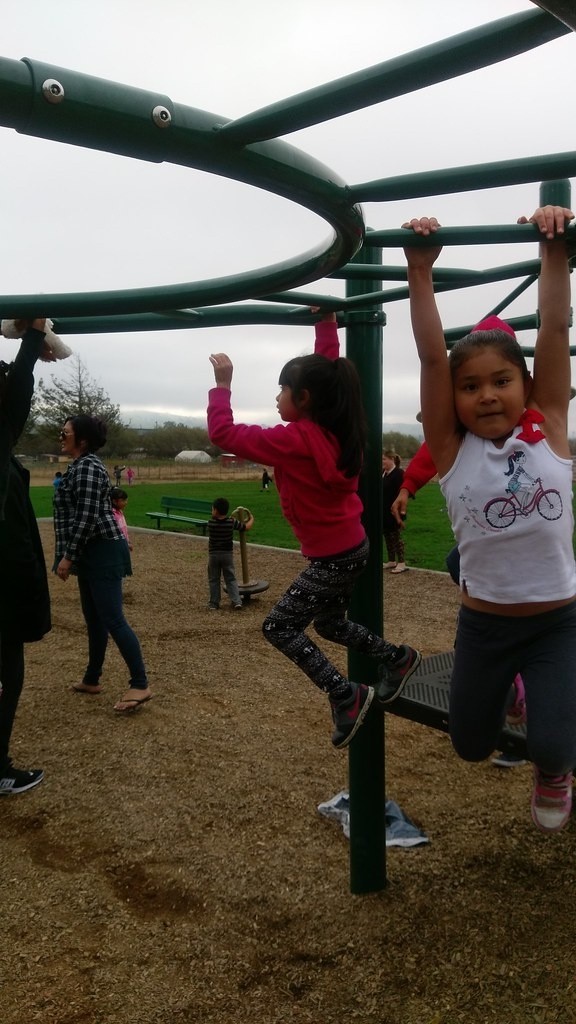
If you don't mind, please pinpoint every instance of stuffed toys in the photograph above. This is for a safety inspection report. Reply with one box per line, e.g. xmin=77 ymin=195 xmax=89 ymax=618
xmin=1 ymin=312 xmax=73 ymax=363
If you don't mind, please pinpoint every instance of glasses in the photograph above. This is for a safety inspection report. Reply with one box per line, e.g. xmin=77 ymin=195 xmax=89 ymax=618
xmin=59 ymin=430 xmax=76 ymax=437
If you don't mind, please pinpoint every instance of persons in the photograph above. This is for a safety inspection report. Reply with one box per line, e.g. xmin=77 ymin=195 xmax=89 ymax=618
xmin=51 ymin=414 xmax=152 ymax=711
xmin=0 ymin=318 xmax=54 ymax=799
xmin=400 ymin=205 xmax=576 ymax=833
xmin=382 ymin=449 xmax=409 ymax=573
xmin=260 ymin=468 xmax=270 ymax=493
xmin=206 ymin=305 xmax=422 ymax=748
xmin=208 ymin=498 xmax=255 ymax=610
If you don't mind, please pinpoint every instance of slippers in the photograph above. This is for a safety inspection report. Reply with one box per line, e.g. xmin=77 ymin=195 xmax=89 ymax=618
xmin=389 ymin=568 xmax=409 ymax=574
xmin=71 ymin=686 xmax=100 ymax=695
xmin=113 ymin=695 xmax=153 ymax=713
xmin=383 ymin=564 xmax=397 ymax=569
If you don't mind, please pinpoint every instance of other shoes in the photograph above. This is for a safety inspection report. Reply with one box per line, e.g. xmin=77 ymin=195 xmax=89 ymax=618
xmin=230 ymin=604 xmax=242 ymax=609
xmin=208 ymin=602 xmax=218 ymax=610
xmin=491 ymin=751 xmax=532 ymax=767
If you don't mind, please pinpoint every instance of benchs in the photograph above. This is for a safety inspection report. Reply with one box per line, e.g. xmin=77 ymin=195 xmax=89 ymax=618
xmin=145 ymin=496 xmax=214 ymax=537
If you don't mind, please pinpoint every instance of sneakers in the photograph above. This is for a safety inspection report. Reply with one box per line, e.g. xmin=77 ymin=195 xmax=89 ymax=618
xmin=328 ymin=681 xmax=375 ymax=749
xmin=374 ymin=644 xmax=422 ymax=705
xmin=0 ymin=763 xmax=44 ymax=796
xmin=530 ymin=764 xmax=575 ymax=835
xmin=505 ymin=672 xmax=527 ymax=724
